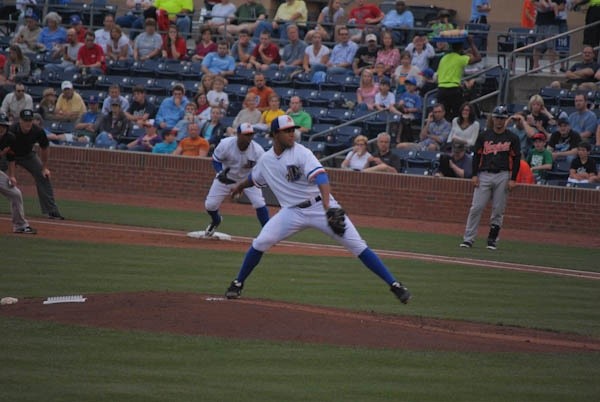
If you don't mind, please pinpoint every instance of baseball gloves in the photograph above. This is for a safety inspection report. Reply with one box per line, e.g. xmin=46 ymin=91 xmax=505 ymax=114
xmin=218 ymin=167 xmax=236 ymax=184
xmin=327 ymin=208 xmax=346 ymax=237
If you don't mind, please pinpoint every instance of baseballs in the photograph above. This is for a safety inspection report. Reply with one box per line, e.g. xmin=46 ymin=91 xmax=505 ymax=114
xmin=231 ymin=191 xmax=240 ymax=200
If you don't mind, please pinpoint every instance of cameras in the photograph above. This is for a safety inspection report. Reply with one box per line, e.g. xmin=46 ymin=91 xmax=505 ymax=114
xmin=433 ymin=153 xmax=451 ymax=167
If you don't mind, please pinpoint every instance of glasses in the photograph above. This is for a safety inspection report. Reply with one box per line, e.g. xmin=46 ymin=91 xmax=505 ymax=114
xmin=16 ymin=89 xmax=25 ymax=92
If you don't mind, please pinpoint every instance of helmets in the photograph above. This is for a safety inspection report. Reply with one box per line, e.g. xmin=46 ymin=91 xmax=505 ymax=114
xmin=0 ymin=111 xmax=9 ymax=126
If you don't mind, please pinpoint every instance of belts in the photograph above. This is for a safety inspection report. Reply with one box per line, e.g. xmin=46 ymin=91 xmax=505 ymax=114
xmin=287 ymin=196 xmax=321 ymax=209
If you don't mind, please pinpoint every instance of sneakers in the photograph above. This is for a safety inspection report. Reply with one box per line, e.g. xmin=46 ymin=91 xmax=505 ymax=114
xmin=47 ymin=213 xmax=64 ymax=220
xmin=391 ymin=281 xmax=411 ymax=304
xmin=459 ymin=242 xmax=472 ymax=248
xmin=12 ymin=227 xmax=37 ymax=234
xmin=225 ymin=281 xmax=243 ymax=299
xmin=487 ymin=241 xmax=497 ymax=250
xmin=204 ymin=216 xmax=222 ymax=237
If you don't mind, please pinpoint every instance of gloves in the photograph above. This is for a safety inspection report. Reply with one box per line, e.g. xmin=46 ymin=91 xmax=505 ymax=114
xmin=327 ymin=207 xmax=346 ymax=235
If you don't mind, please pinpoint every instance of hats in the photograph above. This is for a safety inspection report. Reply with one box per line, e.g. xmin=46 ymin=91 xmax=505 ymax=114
xmin=491 ymin=105 xmax=508 ymax=119
xmin=110 ymin=97 xmax=121 ymax=106
xmin=60 ymin=80 xmax=73 ymax=90
xmin=87 ymin=95 xmax=99 ymax=104
xmin=271 ymin=114 xmax=301 ymax=132
xmin=404 ymin=75 xmax=417 ymax=86
xmin=142 ymin=118 xmax=160 ymax=127
xmin=237 ymin=122 xmax=256 ymax=136
xmin=365 ymin=32 xmax=378 ymax=43
xmin=452 ymin=139 xmax=465 ymax=153
xmin=20 ymin=109 xmax=33 ymax=120
xmin=532 ymin=132 xmax=546 ymax=142
xmin=417 ymin=67 xmax=434 ymax=78
xmin=557 ymin=117 xmax=570 ymax=126
xmin=24 ymin=9 xmax=36 ymax=19
xmin=70 ymin=15 xmax=81 ymax=26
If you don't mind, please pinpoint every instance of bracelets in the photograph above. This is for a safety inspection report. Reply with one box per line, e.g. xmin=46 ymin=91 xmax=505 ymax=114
xmin=346 ymin=151 xmax=354 ymax=160
xmin=145 ymin=54 xmax=150 ymax=59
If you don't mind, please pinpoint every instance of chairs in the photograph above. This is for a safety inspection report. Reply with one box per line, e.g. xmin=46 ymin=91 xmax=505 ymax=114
xmin=391 ymin=88 xmax=600 ymax=190
xmin=391 ymin=2 xmax=570 ymax=71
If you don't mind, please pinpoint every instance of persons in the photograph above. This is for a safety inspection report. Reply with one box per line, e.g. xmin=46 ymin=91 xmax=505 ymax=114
xmin=303 ymin=0 xmax=345 ymax=44
xmin=116 ymin=0 xmax=151 ymax=29
xmin=133 ymin=18 xmax=163 ymax=61
xmin=152 ymin=129 xmax=178 ymax=154
xmin=194 ymin=92 xmax=209 ymax=115
xmin=395 ymin=103 xmax=452 ymax=152
xmin=162 ymin=24 xmax=186 ymax=60
xmin=4 ymin=45 xmax=32 ymax=81
xmin=69 ymin=14 xmax=92 ymax=44
xmin=230 ymin=30 xmax=257 ymax=66
xmin=173 ymin=104 xmax=202 ymax=142
xmin=596 ymin=124 xmax=600 ymax=147
xmin=436 ymin=36 xmax=482 ymax=121
xmin=0 ymin=112 xmax=38 ymax=234
xmin=1 ymin=82 xmax=33 ymax=118
xmin=357 ymin=68 xmax=379 ymax=110
xmin=76 ymin=31 xmax=107 ymax=74
xmin=154 ymin=83 xmax=190 ymax=128
xmin=566 ymin=93 xmax=596 ymax=144
xmin=523 ymin=94 xmax=550 ymax=129
xmin=532 ymin=0 xmax=558 ymax=75
xmin=201 ymin=40 xmax=235 ymax=84
xmin=569 ymin=142 xmax=600 ymax=181
xmin=100 ymin=101 xmax=128 ymax=143
xmin=352 ymin=33 xmax=382 ymax=76
xmin=340 ymin=135 xmax=388 ymax=172
xmin=257 ymin=93 xmax=285 ymax=126
xmin=227 ymin=92 xmax=261 ymax=136
xmin=521 ymin=1 xmax=536 ymax=28
xmin=445 ymin=102 xmax=480 ymax=154
xmin=328 ymin=25 xmax=360 ymax=67
xmin=551 ymin=45 xmax=597 ymax=90
xmin=124 ymin=83 xmax=156 ymax=121
xmin=74 ymin=96 xmax=101 ymax=133
xmin=303 ymin=33 xmax=330 ymax=73
xmin=374 ymin=79 xmax=395 ymax=110
xmin=200 ymin=106 xmax=225 ymax=147
xmin=578 ymin=68 xmax=600 ymax=88
xmin=37 ymin=12 xmax=69 ymax=50
xmin=205 ymin=123 xmax=269 ymax=237
xmin=44 ymin=28 xmax=84 ymax=72
xmin=279 ymin=22 xmax=309 ymax=67
xmin=395 ymin=77 xmax=423 ymax=119
xmin=468 ymin=0 xmax=491 ymax=25
xmin=524 ymin=131 xmax=552 ymax=184
xmin=364 ymin=132 xmax=401 ymax=173
xmin=206 ymin=77 xmax=229 ymax=108
xmin=242 ymin=72 xmax=275 ymax=111
xmin=516 ymin=159 xmax=534 ymax=184
xmin=191 ymin=29 xmax=218 ymax=63
xmin=249 ymin=29 xmax=281 ymax=70
xmin=200 ymin=0 xmax=216 ymax=23
xmin=376 ymin=30 xmax=401 ymax=76
xmin=38 ymin=89 xmax=58 ymax=121
xmin=102 ymin=83 xmax=130 ymax=114
xmin=251 ymin=0 xmax=308 ymax=39
xmin=225 ymin=115 xmax=412 ymax=304
xmin=32 ymin=110 xmax=57 ymax=139
xmin=8 ymin=110 xmax=67 ymax=220
xmin=374 ymin=63 xmax=390 ymax=86
xmin=504 ymin=112 xmax=535 ymax=164
xmin=194 ymin=74 xmax=214 ymax=96
xmin=348 ymin=0 xmax=384 ymax=28
xmin=92 ymin=13 xmax=134 ymax=56
xmin=152 ymin=0 xmax=193 ymax=39
xmin=208 ymin=0 xmax=237 ymax=24
xmin=545 ymin=118 xmax=583 ymax=160
xmin=435 ymin=135 xmax=473 ymax=178
xmin=285 ymin=95 xmax=312 ymax=132
xmin=556 ymin=0 xmax=580 ymax=72
xmin=127 ymin=122 xmax=161 ymax=152
xmin=107 ymin=25 xmax=130 ymax=59
xmin=459 ymin=106 xmax=521 ymax=251
xmin=571 ymin=0 xmax=600 ymax=47
xmin=427 ymin=13 xmax=456 ymax=43
xmin=380 ymin=0 xmax=414 ymax=29
xmin=404 ymin=35 xmax=435 ymax=71
xmin=394 ymin=50 xmax=423 ymax=86
xmin=56 ymin=133 xmax=91 ymax=143
xmin=218 ymin=0 xmax=268 ymax=37
xmin=5 ymin=12 xmax=42 ymax=53
xmin=54 ymin=81 xmax=88 ymax=116
